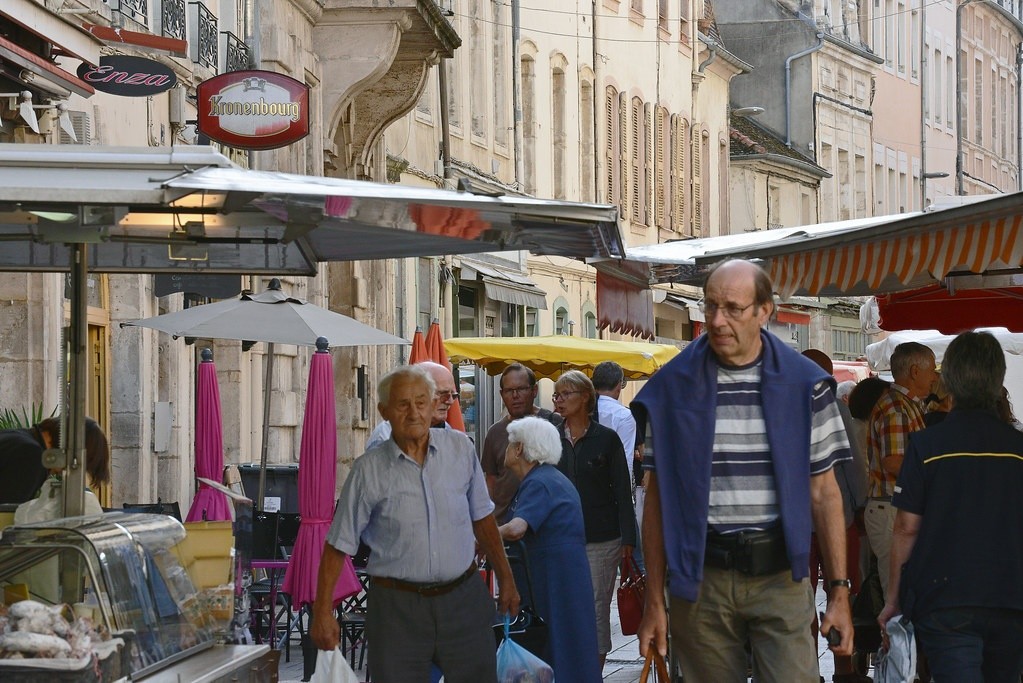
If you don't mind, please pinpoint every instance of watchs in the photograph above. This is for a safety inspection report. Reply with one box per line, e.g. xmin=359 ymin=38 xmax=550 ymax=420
xmin=826 ymin=579 xmax=852 ymax=591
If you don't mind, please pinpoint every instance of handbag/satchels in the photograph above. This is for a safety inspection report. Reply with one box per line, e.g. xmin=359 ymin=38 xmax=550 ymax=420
xmin=874 ymin=615 xmax=919 ymax=683
xmin=617 ymin=553 xmax=646 ymax=637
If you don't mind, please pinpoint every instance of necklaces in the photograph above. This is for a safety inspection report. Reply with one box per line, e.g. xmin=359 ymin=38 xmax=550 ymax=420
xmin=571 ymin=423 xmax=589 ymax=440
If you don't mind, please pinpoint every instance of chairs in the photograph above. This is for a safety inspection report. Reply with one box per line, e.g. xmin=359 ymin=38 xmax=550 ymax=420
xmin=124 ymin=501 xmax=369 ymax=683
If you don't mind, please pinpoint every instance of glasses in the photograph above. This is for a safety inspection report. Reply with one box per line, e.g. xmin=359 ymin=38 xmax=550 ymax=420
xmin=502 ymin=386 xmax=530 ymax=395
xmin=438 ymin=392 xmax=459 ymax=404
xmin=696 ymin=294 xmax=759 ymax=318
xmin=552 ymin=390 xmax=580 ymax=400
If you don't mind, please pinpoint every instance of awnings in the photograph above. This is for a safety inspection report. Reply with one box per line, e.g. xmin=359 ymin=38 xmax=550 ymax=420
xmin=460 ymin=260 xmax=547 ymax=310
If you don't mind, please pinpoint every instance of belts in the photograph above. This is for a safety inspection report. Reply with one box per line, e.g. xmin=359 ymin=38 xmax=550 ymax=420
xmin=371 ymin=560 xmax=479 ymax=594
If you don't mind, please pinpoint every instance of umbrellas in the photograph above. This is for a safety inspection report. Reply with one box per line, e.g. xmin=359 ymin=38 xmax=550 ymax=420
xmin=186 ymin=346 xmax=233 ymax=522
xmin=120 ymin=279 xmax=413 ymax=510
xmin=441 ymin=332 xmax=681 ymax=383
xmin=280 ymin=337 xmax=364 ymax=610
xmin=409 ymin=318 xmax=465 ymax=430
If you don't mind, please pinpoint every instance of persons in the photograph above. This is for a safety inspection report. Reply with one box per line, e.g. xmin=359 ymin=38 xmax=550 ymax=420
xmin=311 ymin=366 xmax=521 ymax=683
xmin=796 ymin=343 xmax=952 ymax=683
xmin=638 ymin=259 xmax=856 ymax=683
xmin=0 ymin=416 xmax=111 ymax=514
xmin=365 ymin=360 xmax=651 ymax=683
xmin=876 ymin=331 xmax=1023 ymax=683
